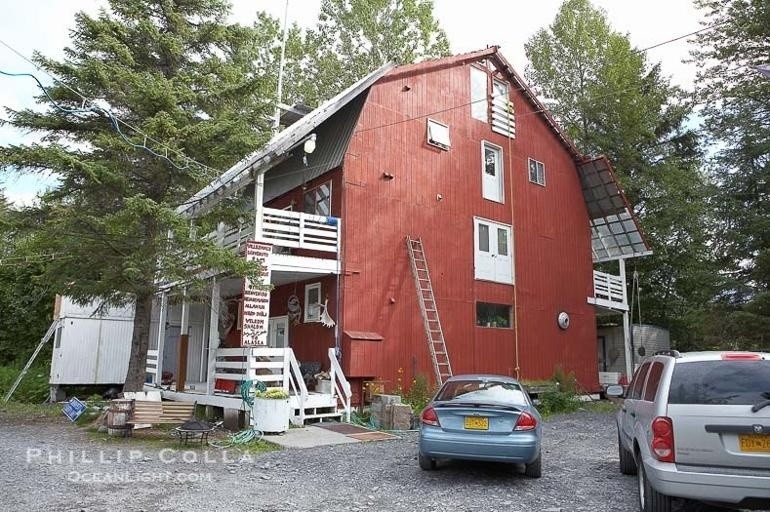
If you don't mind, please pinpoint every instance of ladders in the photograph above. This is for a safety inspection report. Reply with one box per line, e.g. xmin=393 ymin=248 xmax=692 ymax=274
xmin=4 ymin=316 xmax=61 ymax=404
xmin=403 ymin=236 xmax=454 ymax=386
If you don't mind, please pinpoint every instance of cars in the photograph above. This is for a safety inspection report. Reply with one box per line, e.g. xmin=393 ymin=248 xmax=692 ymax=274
xmin=606 ymin=349 xmax=770 ymax=512
xmin=419 ymin=373 xmax=542 ymax=478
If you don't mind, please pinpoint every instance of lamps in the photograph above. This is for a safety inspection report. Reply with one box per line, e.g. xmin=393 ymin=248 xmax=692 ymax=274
xmin=286 ymin=132 xmax=318 ymax=156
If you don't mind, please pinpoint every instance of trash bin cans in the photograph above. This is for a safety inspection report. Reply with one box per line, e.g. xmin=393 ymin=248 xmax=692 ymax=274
xmin=107 ymin=399 xmax=131 ymax=436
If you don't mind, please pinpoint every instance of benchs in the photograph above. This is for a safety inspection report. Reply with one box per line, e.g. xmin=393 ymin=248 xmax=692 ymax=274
xmin=125 ymin=400 xmax=196 ymax=442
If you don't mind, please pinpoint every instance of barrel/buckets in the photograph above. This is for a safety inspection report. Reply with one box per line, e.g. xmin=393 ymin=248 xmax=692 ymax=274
xmin=107 ymin=398 xmax=136 ymax=438
xmin=315 ymin=379 xmax=331 ymax=392
